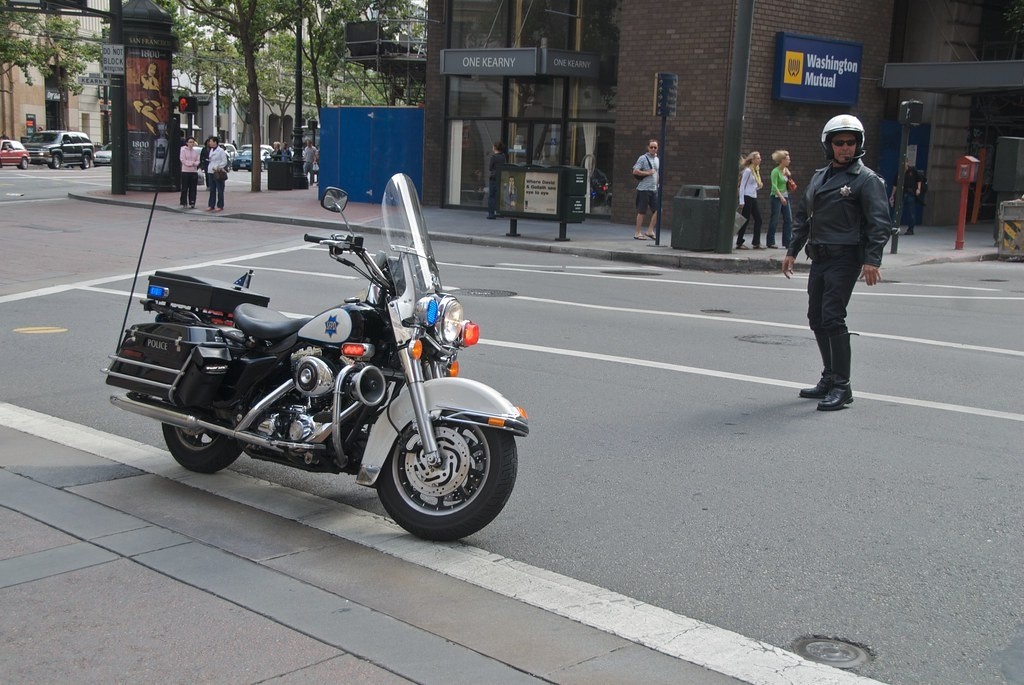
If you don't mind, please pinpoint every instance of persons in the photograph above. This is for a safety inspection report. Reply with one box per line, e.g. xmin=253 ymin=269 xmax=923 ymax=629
xmin=890 ymin=158 xmax=921 ymax=235
xmin=270 ymin=139 xmax=320 ymax=186
xmin=0 ymin=131 xmax=9 ymax=140
xmin=42 ymin=134 xmax=71 ymax=144
xmin=633 ymin=138 xmax=659 ymax=240
xmin=180 ymin=137 xmax=227 ymax=212
xmin=35 ymin=127 xmax=44 ymax=142
xmin=767 ymin=150 xmax=794 ymax=249
xmin=782 ymin=114 xmax=892 ymax=410
xmin=736 ymin=151 xmax=766 ymax=250
xmin=232 ymin=140 xmax=237 ymax=150
xmin=3 ymin=143 xmax=13 ymax=151
xmin=487 ymin=140 xmax=506 ymax=219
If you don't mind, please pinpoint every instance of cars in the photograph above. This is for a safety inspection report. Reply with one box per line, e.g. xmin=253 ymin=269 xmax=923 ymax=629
xmin=93 ymin=142 xmax=276 ymax=172
xmin=233 ymin=146 xmax=274 ymax=172
xmin=22 ymin=130 xmax=93 ymax=169
xmin=0 ymin=140 xmax=31 ymax=169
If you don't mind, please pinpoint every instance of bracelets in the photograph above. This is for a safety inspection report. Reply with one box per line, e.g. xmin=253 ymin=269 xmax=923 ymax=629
xmin=918 ymin=188 xmax=920 ymax=191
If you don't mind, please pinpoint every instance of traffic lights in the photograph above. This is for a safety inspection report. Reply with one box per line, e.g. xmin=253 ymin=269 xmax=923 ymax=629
xmin=179 ymin=97 xmax=198 ymax=114
xmin=899 ymin=99 xmax=924 ymax=126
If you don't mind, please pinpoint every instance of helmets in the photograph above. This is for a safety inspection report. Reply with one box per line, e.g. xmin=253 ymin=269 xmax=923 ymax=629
xmin=821 ymin=115 xmax=865 ymax=162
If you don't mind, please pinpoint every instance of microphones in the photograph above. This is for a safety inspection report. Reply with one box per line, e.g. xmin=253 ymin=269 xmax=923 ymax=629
xmin=845 ymin=150 xmax=866 ymax=161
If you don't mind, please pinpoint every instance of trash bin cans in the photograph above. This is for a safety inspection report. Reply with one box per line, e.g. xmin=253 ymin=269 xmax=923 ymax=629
xmin=671 ymin=184 xmax=720 ymax=254
xmin=267 ymin=155 xmax=292 ymax=191
xmin=997 ymin=198 xmax=1024 ymax=260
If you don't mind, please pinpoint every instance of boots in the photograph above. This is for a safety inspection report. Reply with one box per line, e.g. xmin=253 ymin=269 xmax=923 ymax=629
xmin=799 ymin=334 xmax=831 ymax=397
xmin=817 ymin=333 xmax=859 ymax=409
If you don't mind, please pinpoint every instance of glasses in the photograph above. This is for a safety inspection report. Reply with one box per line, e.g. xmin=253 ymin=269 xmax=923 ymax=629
xmin=832 ymin=140 xmax=857 ymax=146
xmin=650 ymin=147 xmax=656 ymax=149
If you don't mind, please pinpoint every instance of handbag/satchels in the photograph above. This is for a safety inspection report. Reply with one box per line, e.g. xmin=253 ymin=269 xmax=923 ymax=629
xmin=213 ymin=172 xmax=228 ymax=181
xmin=733 ymin=207 xmax=747 ymax=235
xmin=632 ymin=155 xmax=652 ymax=180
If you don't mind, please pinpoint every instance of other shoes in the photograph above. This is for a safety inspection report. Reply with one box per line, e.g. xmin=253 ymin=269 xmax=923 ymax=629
xmin=736 ymin=245 xmax=749 ymax=249
xmin=634 ymin=236 xmax=647 ymax=240
xmin=753 ymin=245 xmax=767 ymax=248
xmin=786 ymin=178 xmax=797 ymax=193
xmin=905 ymin=230 xmax=914 ymax=235
xmin=645 ymin=233 xmax=656 ymax=239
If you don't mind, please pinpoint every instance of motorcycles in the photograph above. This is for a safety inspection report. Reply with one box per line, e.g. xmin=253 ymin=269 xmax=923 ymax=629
xmin=106 ymin=171 xmax=531 ymax=542
